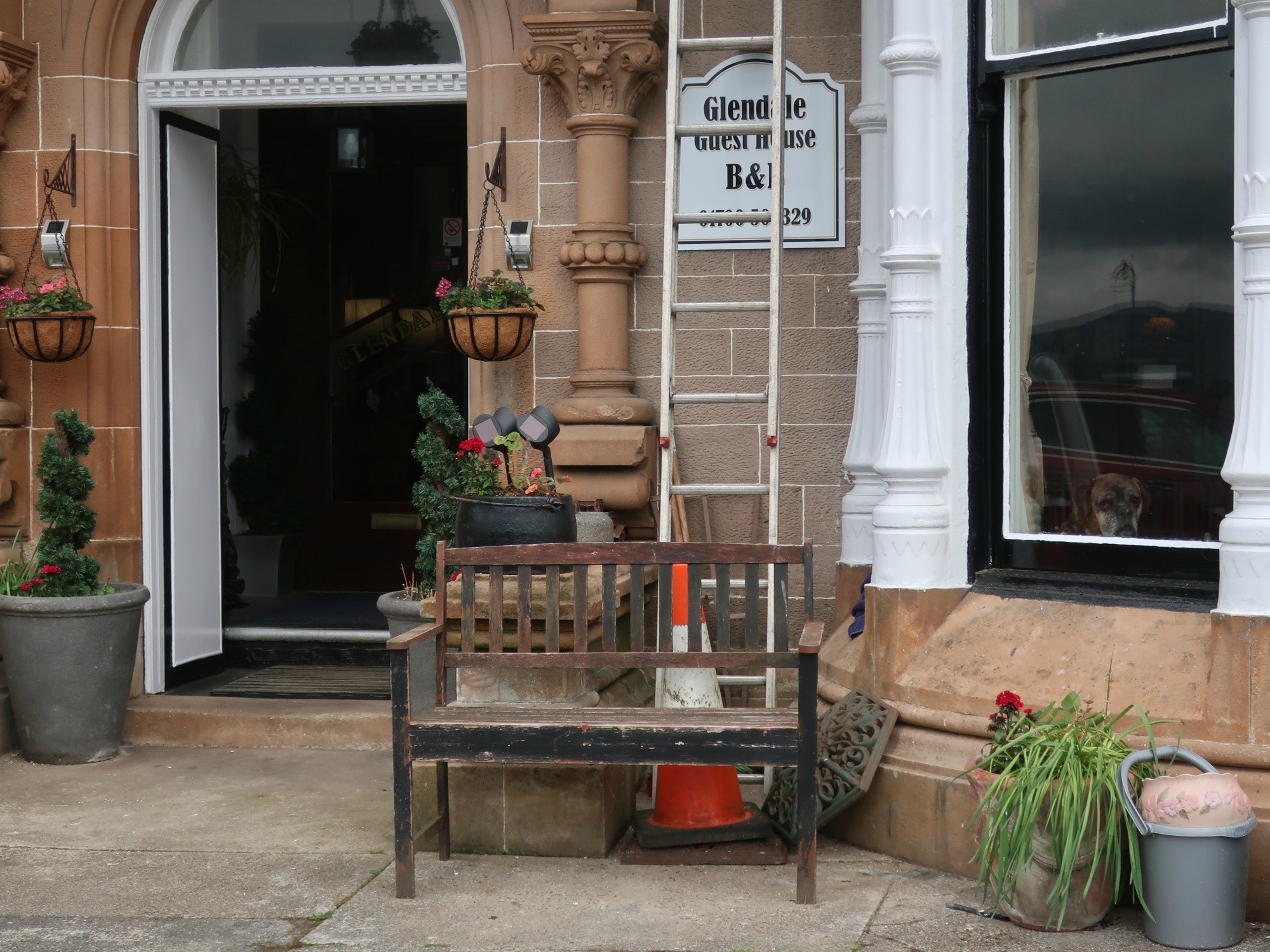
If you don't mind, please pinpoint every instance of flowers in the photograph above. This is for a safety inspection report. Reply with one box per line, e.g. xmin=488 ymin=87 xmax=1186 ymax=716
xmin=455 ymin=431 xmax=559 ymax=497
xmin=1 ymin=408 xmax=119 ymax=599
xmin=966 ymin=686 xmax=1189 ymax=929
xmin=0 ymin=275 xmax=92 ymax=317
xmin=434 ymin=270 xmax=547 ymax=315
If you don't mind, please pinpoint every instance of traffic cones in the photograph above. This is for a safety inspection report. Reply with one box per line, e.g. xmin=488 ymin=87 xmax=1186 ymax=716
xmin=630 ymin=567 xmax=776 ymax=850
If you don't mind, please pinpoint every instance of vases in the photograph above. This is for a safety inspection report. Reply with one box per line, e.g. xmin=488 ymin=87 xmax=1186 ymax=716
xmin=10 ymin=311 xmax=98 ymax=365
xmin=0 ymin=581 xmax=153 ymax=765
xmin=446 ymin=307 xmax=539 ymax=362
xmin=455 ymin=497 xmax=579 ymax=574
xmin=962 ymin=757 xmax=1119 ymax=935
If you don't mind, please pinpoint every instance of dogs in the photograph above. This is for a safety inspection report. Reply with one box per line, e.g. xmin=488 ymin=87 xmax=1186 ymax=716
xmin=1051 ymin=470 xmax=1149 ymax=538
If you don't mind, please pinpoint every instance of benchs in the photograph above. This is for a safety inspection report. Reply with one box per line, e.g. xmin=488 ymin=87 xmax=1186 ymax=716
xmin=386 ymin=540 xmax=827 ymax=908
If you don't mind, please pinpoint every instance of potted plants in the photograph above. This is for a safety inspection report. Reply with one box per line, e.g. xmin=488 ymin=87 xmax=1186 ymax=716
xmin=376 ymin=382 xmax=467 ymax=635
